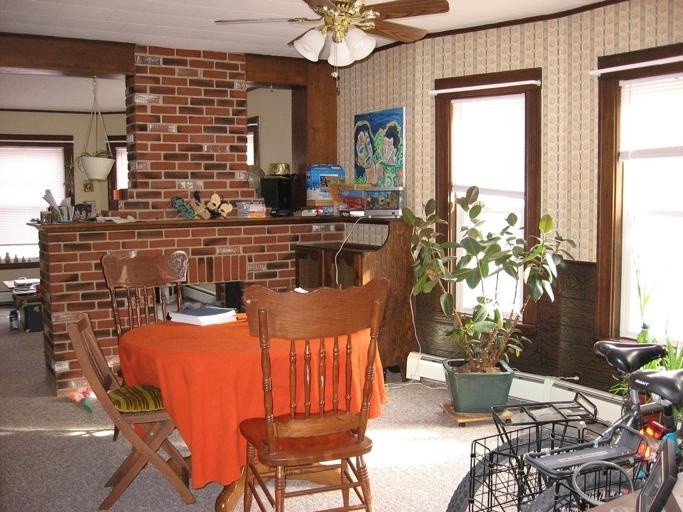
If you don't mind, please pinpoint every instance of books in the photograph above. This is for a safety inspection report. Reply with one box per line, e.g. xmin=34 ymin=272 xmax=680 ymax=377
xmin=168 ymin=305 xmax=238 ymax=326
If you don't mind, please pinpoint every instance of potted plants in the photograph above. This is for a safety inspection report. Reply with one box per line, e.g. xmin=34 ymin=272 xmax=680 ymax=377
xmin=403 ymin=186 xmax=577 ymax=414
xmin=603 ymin=328 xmax=682 ymax=434
xmin=64 ymin=76 xmax=117 ymax=180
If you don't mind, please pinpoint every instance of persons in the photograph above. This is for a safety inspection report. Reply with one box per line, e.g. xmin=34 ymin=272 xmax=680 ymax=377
xmin=379 ymin=122 xmax=400 ymax=166
xmin=353 ymin=121 xmax=402 ymax=188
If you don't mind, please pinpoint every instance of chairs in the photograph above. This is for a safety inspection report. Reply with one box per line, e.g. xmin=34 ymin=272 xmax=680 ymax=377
xmin=235 ymin=276 xmax=390 ymax=511
xmin=100 ymin=251 xmax=192 ymax=443
xmin=67 ymin=313 xmax=199 ymax=509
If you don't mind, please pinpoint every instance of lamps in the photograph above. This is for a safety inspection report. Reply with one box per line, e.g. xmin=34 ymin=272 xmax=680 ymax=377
xmin=294 ymin=24 xmax=378 ymax=66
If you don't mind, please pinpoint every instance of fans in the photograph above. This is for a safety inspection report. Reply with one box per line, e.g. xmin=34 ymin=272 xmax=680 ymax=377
xmin=214 ymin=0 xmax=449 ymax=46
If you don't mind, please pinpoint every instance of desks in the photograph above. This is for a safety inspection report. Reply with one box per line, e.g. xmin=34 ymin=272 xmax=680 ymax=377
xmin=118 ymin=311 xmax=388 ymax=512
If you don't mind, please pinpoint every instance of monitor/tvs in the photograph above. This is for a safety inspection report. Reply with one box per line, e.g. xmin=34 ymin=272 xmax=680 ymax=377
xmin=261 ymin=174 xmax=306 ymax=217
xmin=636 ymin=439 xmax=683 ymax=512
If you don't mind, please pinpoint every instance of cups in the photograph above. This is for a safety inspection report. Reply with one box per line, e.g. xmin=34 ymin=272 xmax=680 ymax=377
xmin=236 ymin=202 xmax=249 ymax=217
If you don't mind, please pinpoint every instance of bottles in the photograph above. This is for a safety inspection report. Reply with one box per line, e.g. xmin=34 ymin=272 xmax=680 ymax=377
xmin=8 ymin=309 xmax=18 ymax=330
xmin=248 ymin=202 xmax=254 ymax=217
